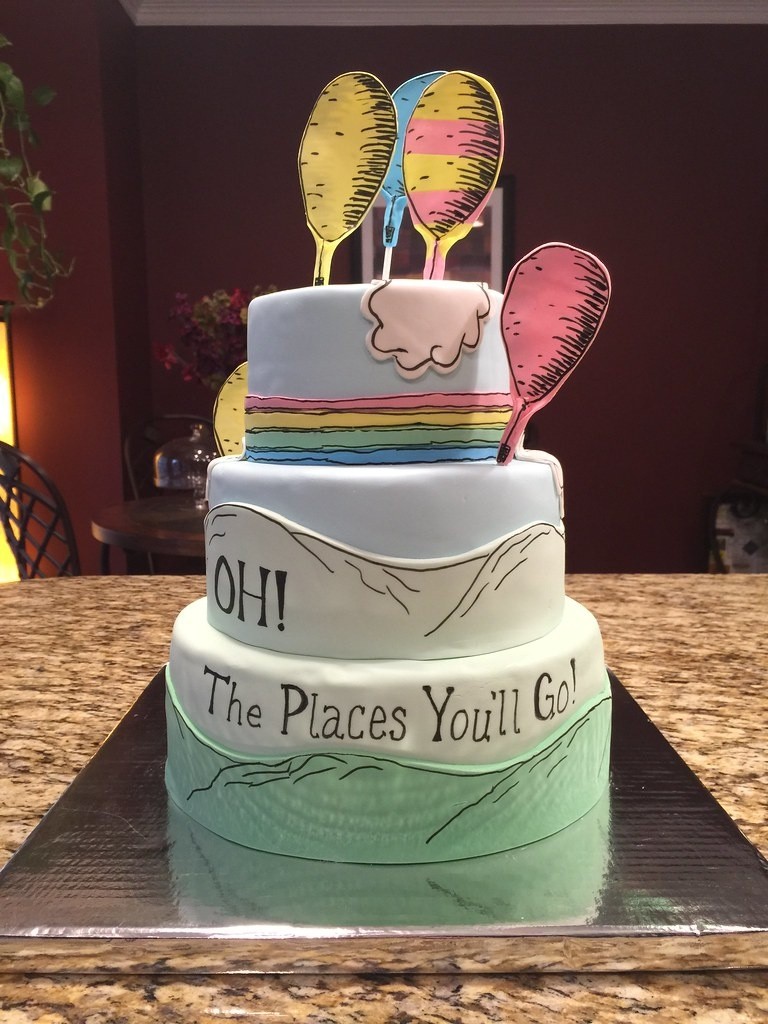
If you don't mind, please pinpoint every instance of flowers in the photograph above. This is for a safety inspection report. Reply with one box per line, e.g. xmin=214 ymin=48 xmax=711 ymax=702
xmin=156 ymin=283 xmax=278 ymax=389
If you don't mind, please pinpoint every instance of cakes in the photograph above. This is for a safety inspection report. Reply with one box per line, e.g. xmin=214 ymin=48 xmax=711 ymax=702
xmin=164 ymin=66 xmax=612 ymax=864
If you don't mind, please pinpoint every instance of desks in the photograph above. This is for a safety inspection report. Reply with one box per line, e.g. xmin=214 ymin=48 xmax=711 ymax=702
xmin=91 ymin=495 xmax=209 ymax=577
xmin=0 ymin=572 xmax=768 ymax=1024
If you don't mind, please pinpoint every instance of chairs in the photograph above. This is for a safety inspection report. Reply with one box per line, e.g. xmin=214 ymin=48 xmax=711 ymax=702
xmin=0 ymin=443 xmax=81 ymax=579
xmin=125 ymin=414 xmax=213 ymax=573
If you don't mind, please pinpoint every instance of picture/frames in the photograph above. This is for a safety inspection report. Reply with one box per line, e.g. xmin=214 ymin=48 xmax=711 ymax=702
xmin=350 ymin=174 xmax=517 ymax=293
